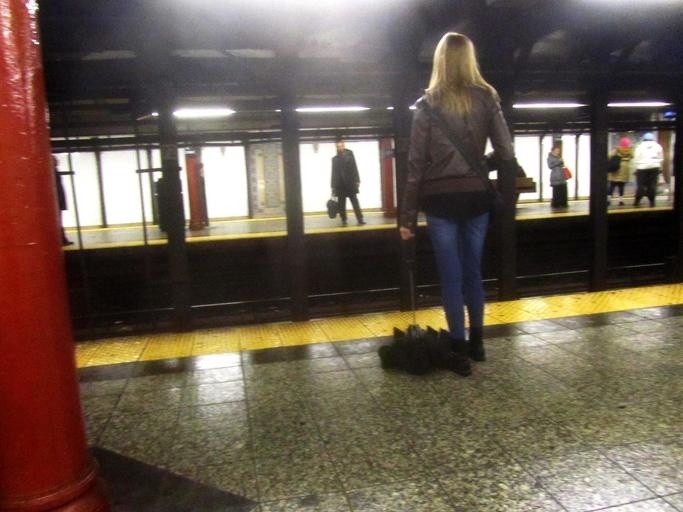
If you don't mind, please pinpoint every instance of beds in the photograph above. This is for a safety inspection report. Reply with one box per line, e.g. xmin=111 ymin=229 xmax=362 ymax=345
xmin=377 ymin=258 xmax=452 ymax=376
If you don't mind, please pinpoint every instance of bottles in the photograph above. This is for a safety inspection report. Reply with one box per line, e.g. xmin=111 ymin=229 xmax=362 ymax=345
xmin=620 ymin=136 xmax=632 ymax=146
xmin=643 ymin=133 xmax=656 ymax=141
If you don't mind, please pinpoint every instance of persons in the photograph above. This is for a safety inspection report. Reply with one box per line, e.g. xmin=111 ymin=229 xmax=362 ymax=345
xmin=52 ymin=156 xmax=75 ymax=246
xmin=330 ymin=140 xmax=367 ymax=226
xmin=399 ymin=33 xmax=515 ymax=377
xmin=548 ymin=146 xmax=567 ymax=207
xmin=607 ymin=133 xmax=664 ymax=207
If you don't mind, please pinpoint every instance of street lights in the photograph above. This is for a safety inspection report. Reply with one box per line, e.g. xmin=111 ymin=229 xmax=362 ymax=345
xmin=326 ymin=197 xmax=339 ymax=218
xmin=561 ymin=165 xmax=572 ymax=180
xmin=488 ymin=189 xmax=507 ymax=219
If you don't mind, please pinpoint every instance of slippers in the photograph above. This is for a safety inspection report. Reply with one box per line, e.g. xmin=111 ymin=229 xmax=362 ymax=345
xmin=607 ymin=151 xmax=622 ymax=172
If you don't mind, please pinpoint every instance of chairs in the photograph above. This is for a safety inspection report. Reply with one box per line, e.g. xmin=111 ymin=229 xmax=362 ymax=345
xmin=465 ymin=337 xmax=486 ymax=361
xmin=606 ymin=201 xmax=624 ymax=205
xmin=448 ymin=353 xmax=473 ymax=376
